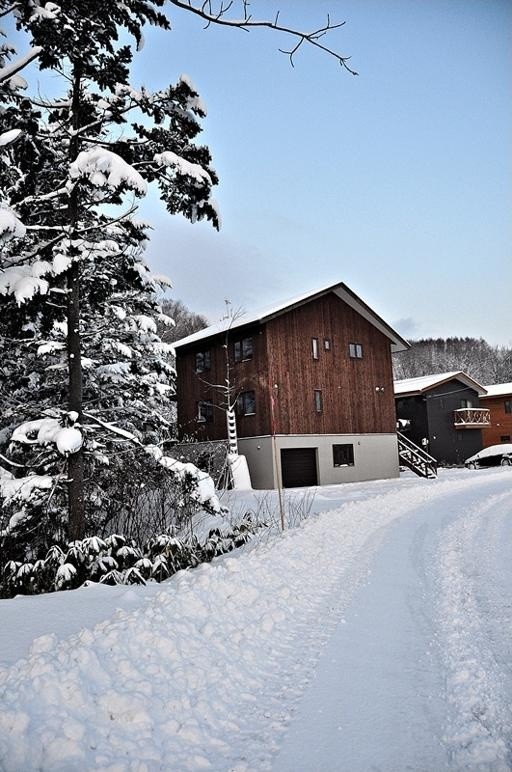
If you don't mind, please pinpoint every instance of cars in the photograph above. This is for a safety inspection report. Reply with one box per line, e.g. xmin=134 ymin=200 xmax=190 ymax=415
xmin=464 ymin=444 xmax=512 ymax=470
